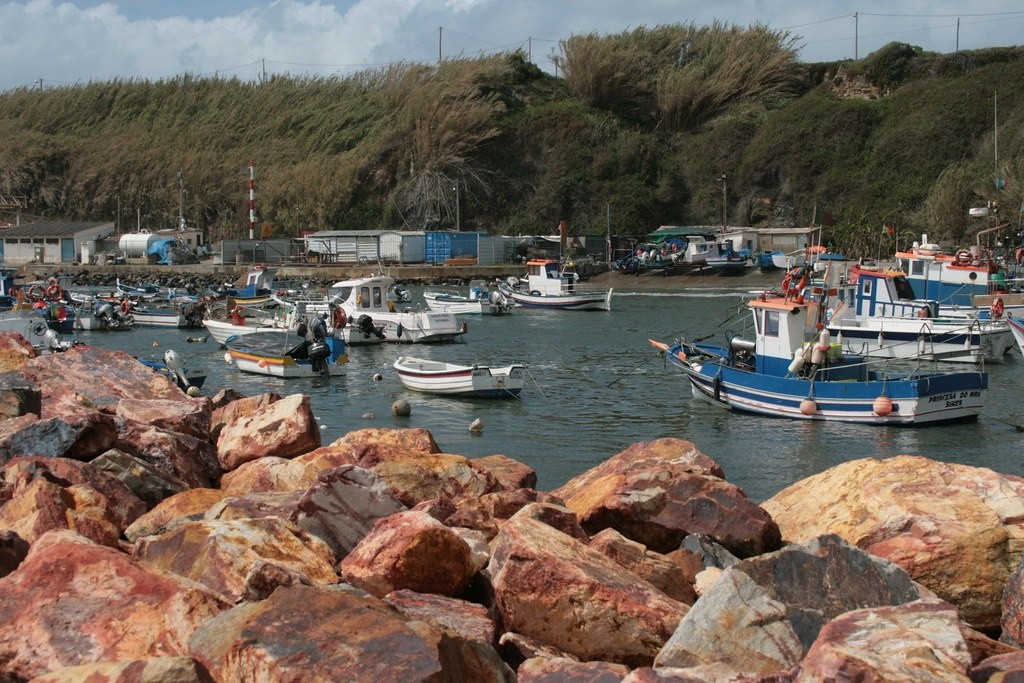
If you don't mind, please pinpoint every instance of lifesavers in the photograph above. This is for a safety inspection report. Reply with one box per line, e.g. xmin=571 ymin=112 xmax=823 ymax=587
xmin=276 ymin=291 xmax=284 ymax=296
xmin=121 ymin=301 xmax=129 ymax=315
xmin=761 ymin=291 xmax=778 ymax=297
xmin=672 ymin=244 xmax=677 ymax=251
xmin=50 ymin=279 xmax=57 ymax=286
xmin=29 ymin=285 xmax=46 ymax=302
xmin=1015 ymin=248 xmax=1022 ymax=263
xmin=662 ymin=249 xmax=666 ymax=255
xmin=993 ymin=297 xmax=1004 ymax=319
xmin=331 ymin=307 xmax=347 ymax=330
xmin=46 ymin=284 xmax=63 ymax=302
xmin=955 ymin=249 xmax=973 ymax=266
xmin=780 ymin=267 xmax=807 ymax=294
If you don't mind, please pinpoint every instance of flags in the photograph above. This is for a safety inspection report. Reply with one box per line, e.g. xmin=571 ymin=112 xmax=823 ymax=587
xmin=998 ymin=176 xmax=1009 ymax=190
xmin=882 ymin=225 xmax=894 ymax=235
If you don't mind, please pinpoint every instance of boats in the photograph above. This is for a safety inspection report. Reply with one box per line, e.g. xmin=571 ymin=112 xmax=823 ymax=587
xmin=392 ymin=356 xmax=530 ymax=399
xmin=0 ymin=233 xmax=1024 ymax=363
xmin=225 ymin=329 xmax=352 ymax=379
xmin=645 ymin=250 xmax=990 ymax=426
